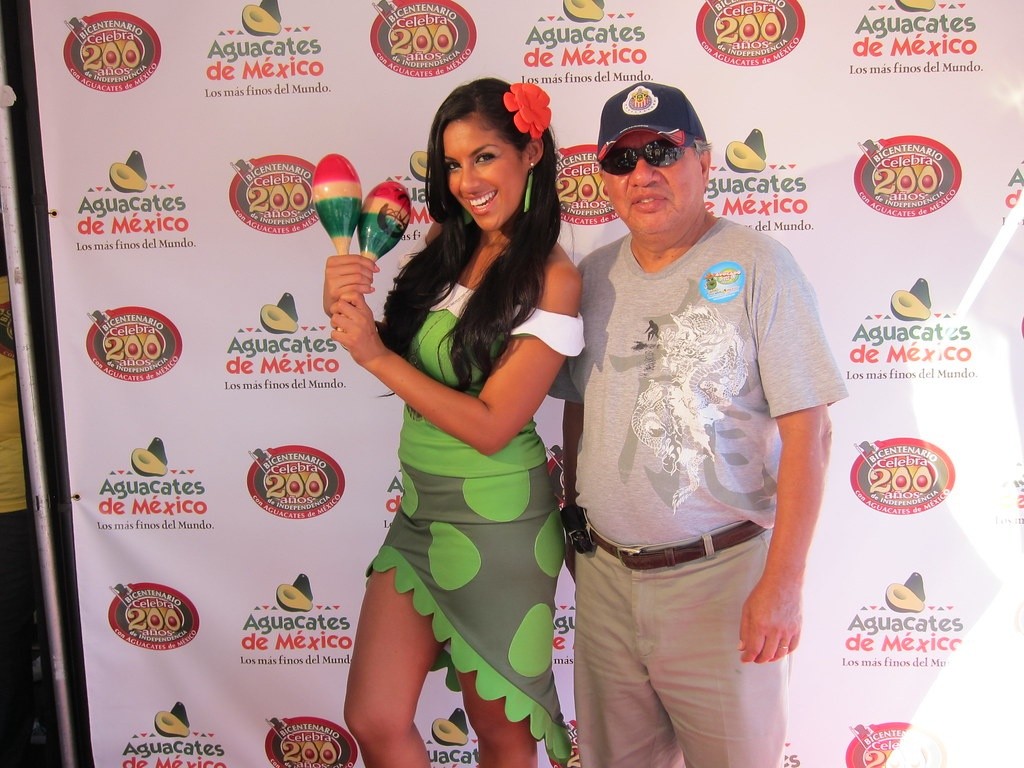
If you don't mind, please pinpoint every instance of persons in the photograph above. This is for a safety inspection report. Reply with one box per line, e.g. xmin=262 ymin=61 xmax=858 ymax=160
xmin=543 ymin=81 xmax=848 ymax=768
xmin=322 ymin=77 xmax=584 ymax=768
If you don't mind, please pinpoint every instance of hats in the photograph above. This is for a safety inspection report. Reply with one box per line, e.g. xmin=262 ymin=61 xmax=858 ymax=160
xmin=597 ymin=82 xmax=706 ymax=159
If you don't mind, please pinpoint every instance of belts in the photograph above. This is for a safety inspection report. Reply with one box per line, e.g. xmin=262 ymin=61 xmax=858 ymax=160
xmin=587 ymin=520 xmax=765 ymax=571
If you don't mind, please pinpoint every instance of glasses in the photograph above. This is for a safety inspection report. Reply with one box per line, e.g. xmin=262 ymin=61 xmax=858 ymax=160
xmin=599 ymin=139 xmax=683 ymax=175
